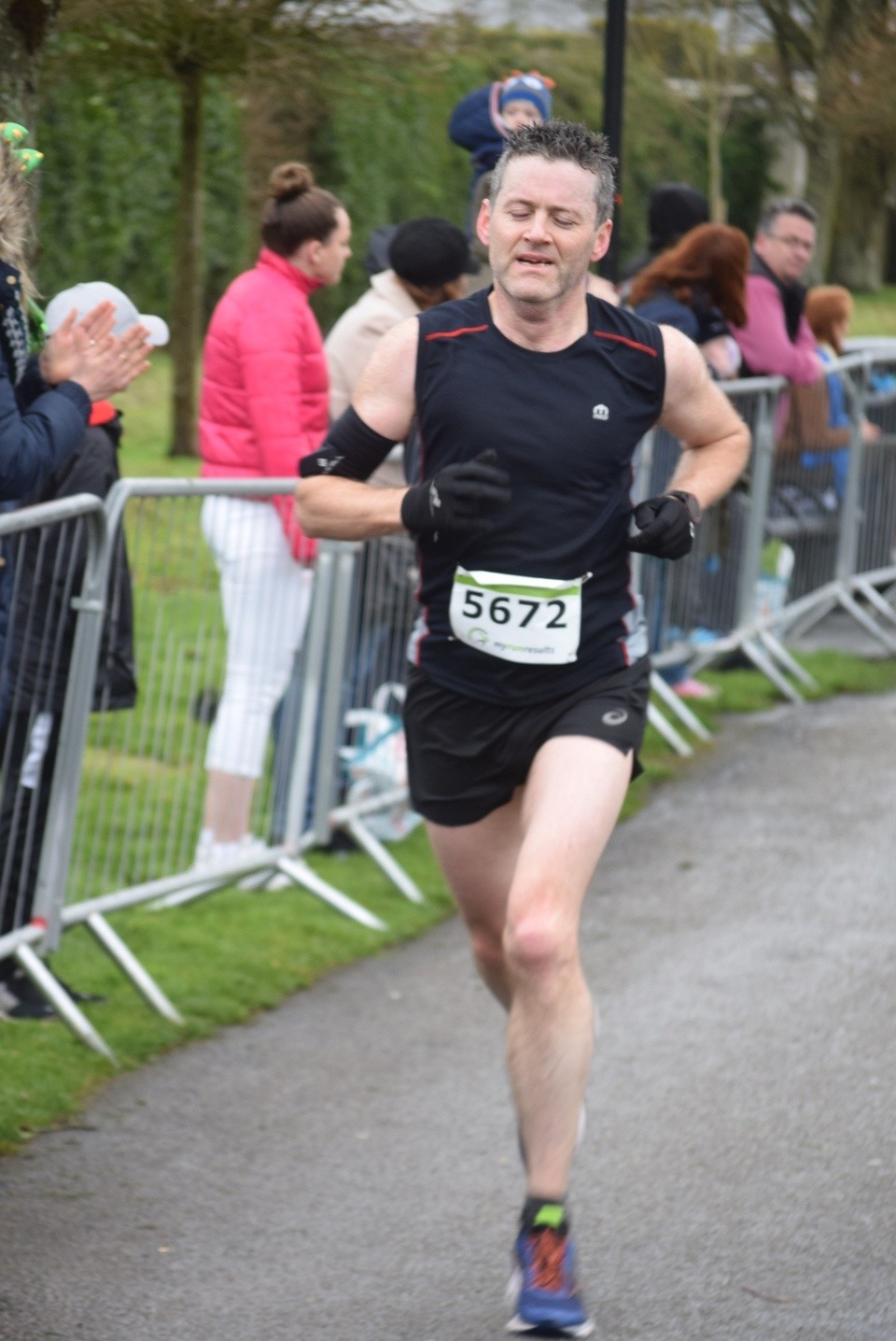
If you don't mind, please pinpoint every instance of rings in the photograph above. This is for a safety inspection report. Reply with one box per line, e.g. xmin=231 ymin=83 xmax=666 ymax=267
xmin=90 ymin=338 xmax=93 ymax=344
xmin=120 ymin=354 xmax=125 ymax=359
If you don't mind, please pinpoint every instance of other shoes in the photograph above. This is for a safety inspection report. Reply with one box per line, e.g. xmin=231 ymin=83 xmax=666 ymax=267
xmin=192 ymin=832 xmax=294 ymax=889
xmin=1 ymin=967 xmax=100 ymax=1023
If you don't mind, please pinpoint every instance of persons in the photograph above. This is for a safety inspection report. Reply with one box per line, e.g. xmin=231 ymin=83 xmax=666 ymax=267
xmin=0 ymin=122 xmax=153 ymax=1020
xmin=269 ymin=219 xmax=479 ymax=855
xmin=295 ymin=120 xmax=751 ymax=1339
xmin=447 ymin=68 xmax=556 ymax=171
xmin=615 ymin=187 xmax=880 ymax=700
xmin=197 ymin=162 xmax=353 ymax=892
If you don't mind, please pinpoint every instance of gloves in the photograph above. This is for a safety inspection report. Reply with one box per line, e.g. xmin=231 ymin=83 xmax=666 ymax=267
xmin=397 ymin=449 xmax=510 ymax=533
xmin=625 ymin=494 xmax=697 ymax=560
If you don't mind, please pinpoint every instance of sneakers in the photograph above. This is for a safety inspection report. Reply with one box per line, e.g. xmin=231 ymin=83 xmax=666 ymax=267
xmin=508 ymin=1207 xmax=595 ymax=1339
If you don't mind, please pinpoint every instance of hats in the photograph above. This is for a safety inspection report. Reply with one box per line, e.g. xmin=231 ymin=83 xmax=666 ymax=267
xmin=645 ymin=185 xmax=708 ymax=240
xmin=392 ymin=218 xmax=482 ymax=283
xmin=41 ymin=280 xmax=169 ymax=350
xmin=499 ymin=75 xmax=553 ymax=122
